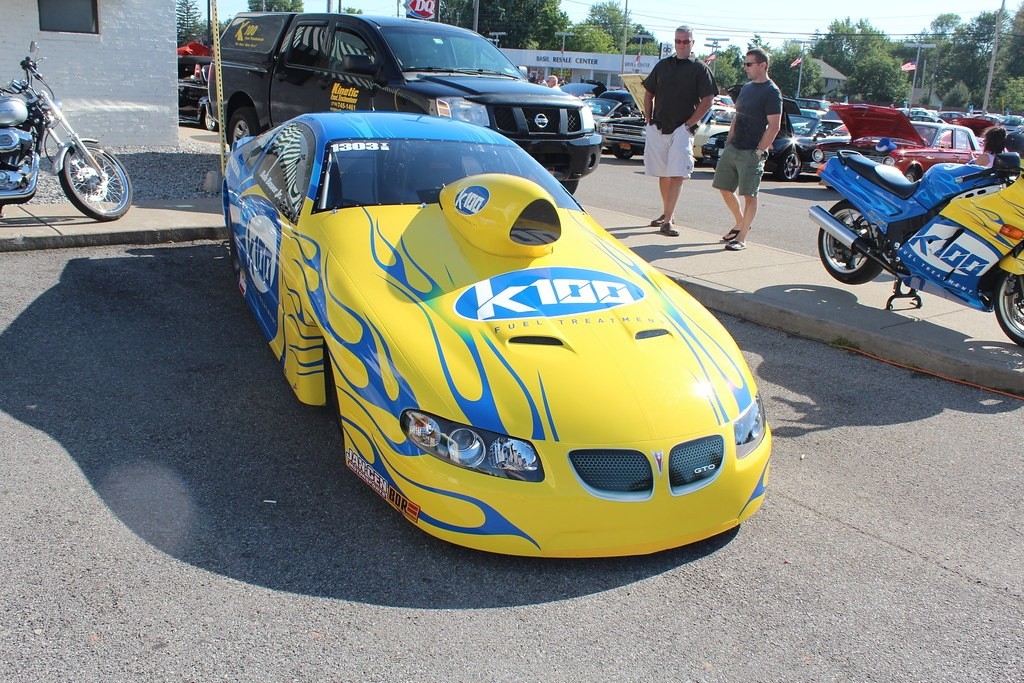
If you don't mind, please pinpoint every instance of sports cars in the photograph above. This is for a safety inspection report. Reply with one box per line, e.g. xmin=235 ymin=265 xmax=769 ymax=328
xmin=221 ymin=111 xmax=773 ymax=558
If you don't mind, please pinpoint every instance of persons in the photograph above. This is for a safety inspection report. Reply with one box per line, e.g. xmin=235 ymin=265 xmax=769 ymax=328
xmin=528 ymin=70 xmax=548 ymax=86
xmin=640 ymin=25 xmax=719 ymax=236
xmin=558 ymin=78 xmax=568 ymax=87
xmin=712 ymin=48 xmax=783 ymax=250
xmin=547 ymin=75 xmax=561 ymax=90
xmin=975 ymin=126 xmax=1007 ymax=167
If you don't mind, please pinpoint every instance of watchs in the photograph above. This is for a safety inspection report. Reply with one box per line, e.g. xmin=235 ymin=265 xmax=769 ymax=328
xmin=685 ymin=124 xmax=689 ymax=130
xmin=757 ymin=150 xmax=763 ymax=157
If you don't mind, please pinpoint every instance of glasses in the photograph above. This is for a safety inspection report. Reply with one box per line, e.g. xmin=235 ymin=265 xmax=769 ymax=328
xmin=675 ymin=38 xmax=694 ymax=45
xmin=744 ymin=61 xmax=765 ymax=66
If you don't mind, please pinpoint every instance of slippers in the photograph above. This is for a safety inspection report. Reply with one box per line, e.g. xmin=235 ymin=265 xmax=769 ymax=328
xmin=723 ymin=225 xmax=751 ymax=240
xmin=725 ymin=239 xmax=746 ymax=250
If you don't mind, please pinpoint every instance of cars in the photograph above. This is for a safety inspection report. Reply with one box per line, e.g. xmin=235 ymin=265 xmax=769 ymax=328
xmin=561 ymin=74 xmax=1024 ymax=192
xmin=178 ymin=55 xmax=219 ymax=132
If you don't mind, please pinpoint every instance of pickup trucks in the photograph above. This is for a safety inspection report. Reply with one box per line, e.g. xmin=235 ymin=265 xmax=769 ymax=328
xmin=207 ymin=10 xmax=603 ymax=196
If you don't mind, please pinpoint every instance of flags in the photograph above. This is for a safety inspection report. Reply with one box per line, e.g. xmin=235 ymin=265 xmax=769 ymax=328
xmin=635 ymin=51 xmax=640 ymax=64
xmin=789 ymin=52 xmax=802 ymax=69
xmin=704 ymin=52 xmax=715 ymax=65
xmin=902 ymin=61 xmax=916 ymax=71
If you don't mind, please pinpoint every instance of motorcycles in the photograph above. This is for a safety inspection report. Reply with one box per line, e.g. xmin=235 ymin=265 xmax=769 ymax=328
xmin=807 ymin=131 xmax=1023 ymax=350
xmin=0 ymin=41 xmax=133 ymax=223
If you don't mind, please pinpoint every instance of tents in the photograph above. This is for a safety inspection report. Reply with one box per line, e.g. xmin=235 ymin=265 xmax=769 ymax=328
xmin=177 ymin=42 xmax=214 ymax=56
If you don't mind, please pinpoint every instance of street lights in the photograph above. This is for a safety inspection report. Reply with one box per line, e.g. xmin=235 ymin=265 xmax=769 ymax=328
xmin=632 ymin=34 xmax=656 ymax=55
xmin=489 ymin=32 xmax=507 ymax=50
xmin=554 ymin=30 xmax=575 ymax=81
xmin=703 ymin=37 xmax=729 ymax=76
xmin=904 ymin=42 xmax=936 ymax=116
xmin=791 ymin=39 xmax=816 ymax=98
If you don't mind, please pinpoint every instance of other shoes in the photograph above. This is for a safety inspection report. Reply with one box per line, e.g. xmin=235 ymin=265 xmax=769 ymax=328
xmin=660 ymin=223 xmax=680 ymax=236
xmin=651 ymin=214 xmax=675 ymax=227
